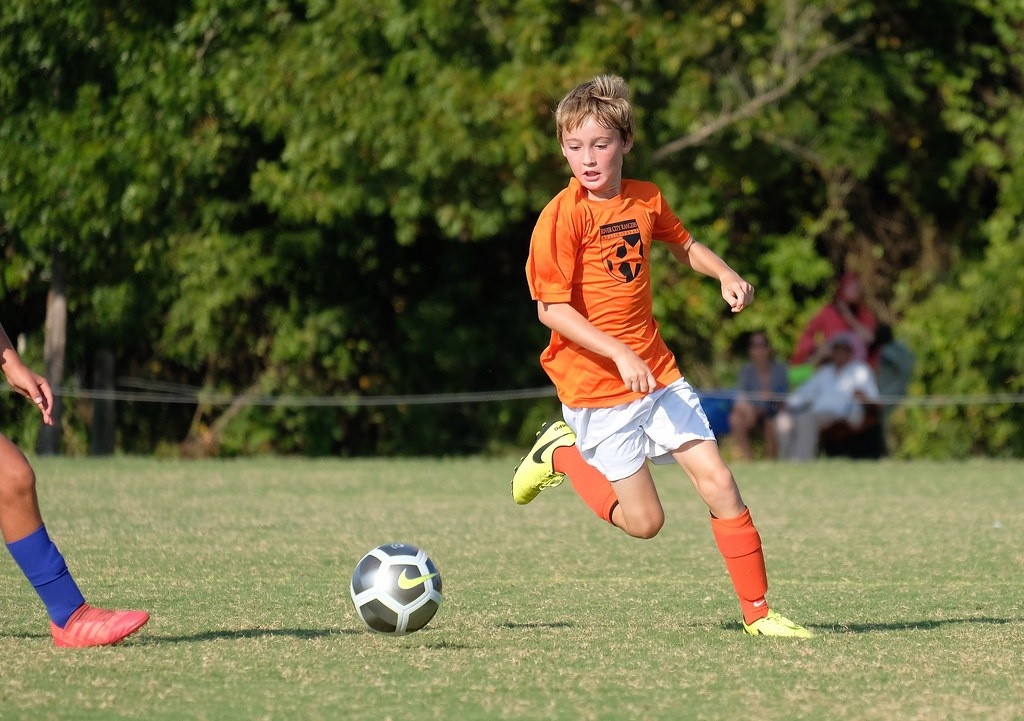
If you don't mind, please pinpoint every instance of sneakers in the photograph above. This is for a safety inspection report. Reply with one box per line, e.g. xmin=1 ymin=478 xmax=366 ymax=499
xmin=512 ymin=419 xmax=576 ymax=505
xmin=742 ymin=609 xmax=815 ymax=640
xmin=48 ymin=605 xmax=150 ymax=646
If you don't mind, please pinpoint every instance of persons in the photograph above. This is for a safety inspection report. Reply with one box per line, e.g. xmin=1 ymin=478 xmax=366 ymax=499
xmin=510 ymin=76 xmax=820 ymax=641
xmin=732 ymin=273 xmax=913 ymax=464
xmin=1 ymin=324 xmax=150 ymax=650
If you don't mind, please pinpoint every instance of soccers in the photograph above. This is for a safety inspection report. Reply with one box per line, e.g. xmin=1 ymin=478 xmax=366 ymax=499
xmin=349 ymin=543 xmax=444 ymax=638
